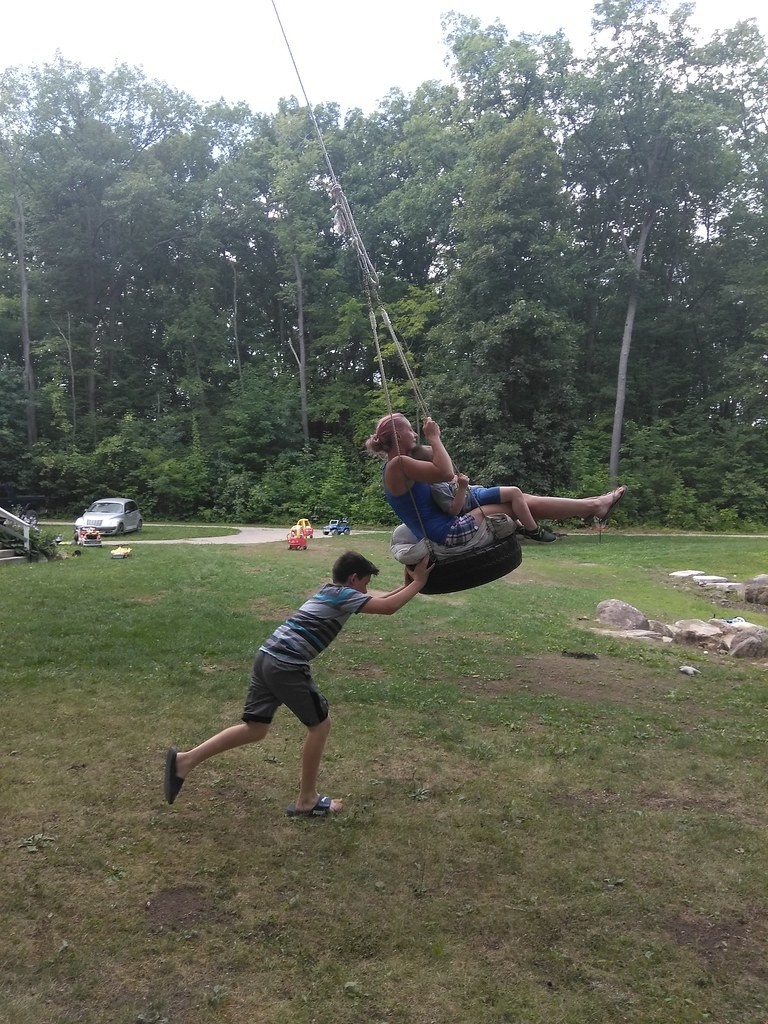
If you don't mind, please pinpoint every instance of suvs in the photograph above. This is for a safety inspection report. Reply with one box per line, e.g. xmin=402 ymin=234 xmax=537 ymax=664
xmin=322 ymin=520 xmax=350 ymax=535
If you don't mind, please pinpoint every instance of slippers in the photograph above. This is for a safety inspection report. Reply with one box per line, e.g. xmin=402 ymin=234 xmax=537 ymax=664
xmin=584 ymin=485 xmax=628 ymax=521
xmin=287 ymin=795 xmax=344 ymax=818
xmin=164 ymin=747 xmax=184 ymax=805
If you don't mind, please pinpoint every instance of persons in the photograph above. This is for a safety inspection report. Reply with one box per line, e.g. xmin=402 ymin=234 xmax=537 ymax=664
xmin=164 ymin=551 xmax=435 ymax=818
xmin=366 ymin=413 xmax=627 ymax=548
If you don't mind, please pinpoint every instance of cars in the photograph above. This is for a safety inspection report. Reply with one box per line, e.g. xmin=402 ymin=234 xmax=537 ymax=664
xmin=73 ymin=497 xmax=143 ymax=540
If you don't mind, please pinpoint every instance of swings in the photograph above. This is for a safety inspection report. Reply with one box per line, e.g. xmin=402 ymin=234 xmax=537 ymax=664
xmin=270 ymin=0 xmax=525 ymax=597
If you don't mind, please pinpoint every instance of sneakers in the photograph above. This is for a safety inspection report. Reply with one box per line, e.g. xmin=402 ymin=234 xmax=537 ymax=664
xmin=524 ymin=526 xmax=556 ymax=543
xmin=515 ymin=525 xmax=526 ymax=535
